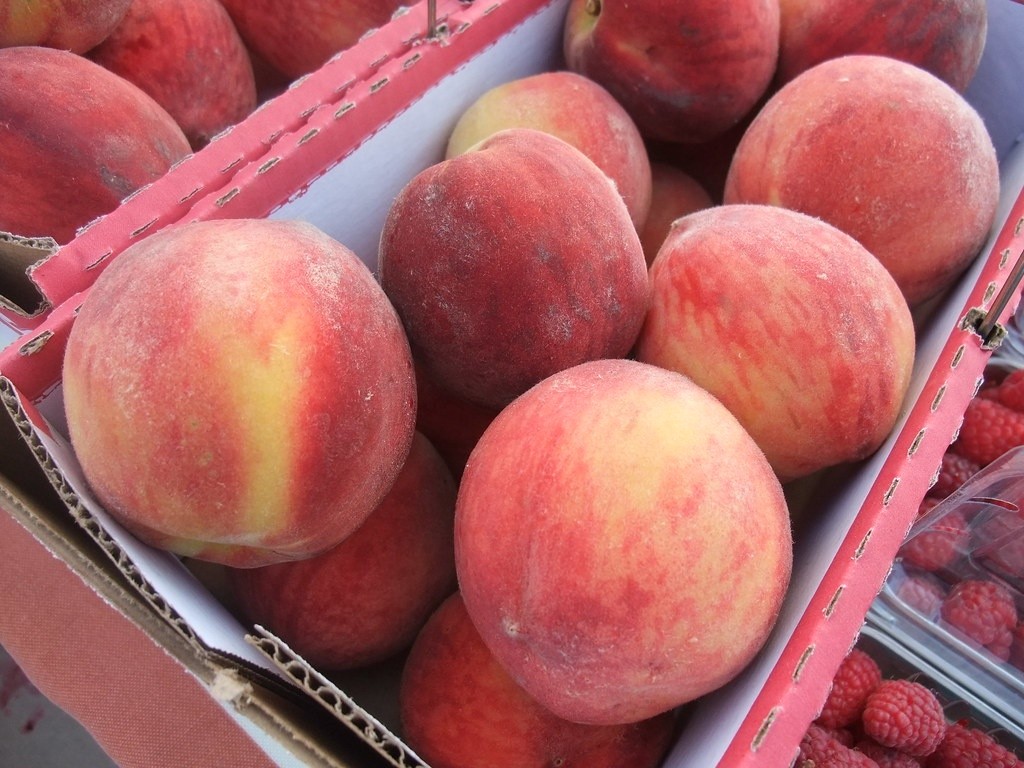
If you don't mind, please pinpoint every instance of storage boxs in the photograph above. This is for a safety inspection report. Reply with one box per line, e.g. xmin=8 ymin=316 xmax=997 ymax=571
xmin=0 ymin=0 xmax=1024 ymax=768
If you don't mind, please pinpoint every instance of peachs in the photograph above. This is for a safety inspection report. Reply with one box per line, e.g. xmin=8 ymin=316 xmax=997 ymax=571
xmin=0 ymin=0 xmax=999 ymax=768
